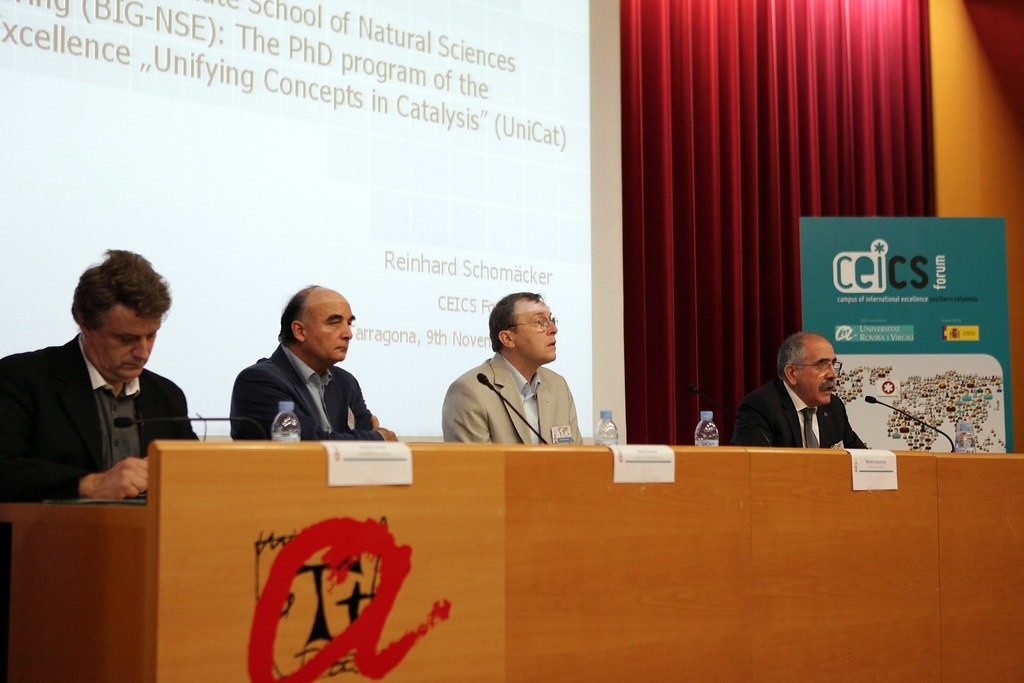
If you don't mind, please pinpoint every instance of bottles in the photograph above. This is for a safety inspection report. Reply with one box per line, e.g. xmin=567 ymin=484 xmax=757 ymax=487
xmin=271 ymin=401 xmax=301 ymax=442
xmin=594 ymin=411 xmax=618 ymax=446
xmin=954 ymin=423 xmax=976 ymax=454
xmin=694 ymin=411 xmax=719 ymax=447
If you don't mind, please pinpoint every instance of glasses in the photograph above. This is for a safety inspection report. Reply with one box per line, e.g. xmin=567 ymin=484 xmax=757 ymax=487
xmin=792 ymin=360 xmax=843 ymax=372
xmin=506 ymin=316 xmax=558 ymax=332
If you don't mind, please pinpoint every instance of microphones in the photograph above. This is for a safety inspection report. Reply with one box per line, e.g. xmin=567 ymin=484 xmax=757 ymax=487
xmin=865 ymin=396 xmax=955 ymax=453
xmin=114 ymin=416 xmax=268 ymax=441
xmin=477 ymin=373 xmax=549 ymax=445
xmin=686 ymin=383 xmax=773 ymax=447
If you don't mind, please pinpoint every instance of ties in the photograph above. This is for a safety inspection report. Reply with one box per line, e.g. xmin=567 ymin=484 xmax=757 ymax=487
xmin=801 ymin=407 xmax=819 ymax=448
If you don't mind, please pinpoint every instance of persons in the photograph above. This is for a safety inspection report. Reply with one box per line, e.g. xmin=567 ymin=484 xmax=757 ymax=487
xmin=0 ymin=249 xmax=201 ymax=682
xmin=730 ymin=331 xmax=869 ymax=448
xmin=441 ymin=292 xmax=583 ymax=443
xmin=228 ymin=283 xmax=397 ymax=442
